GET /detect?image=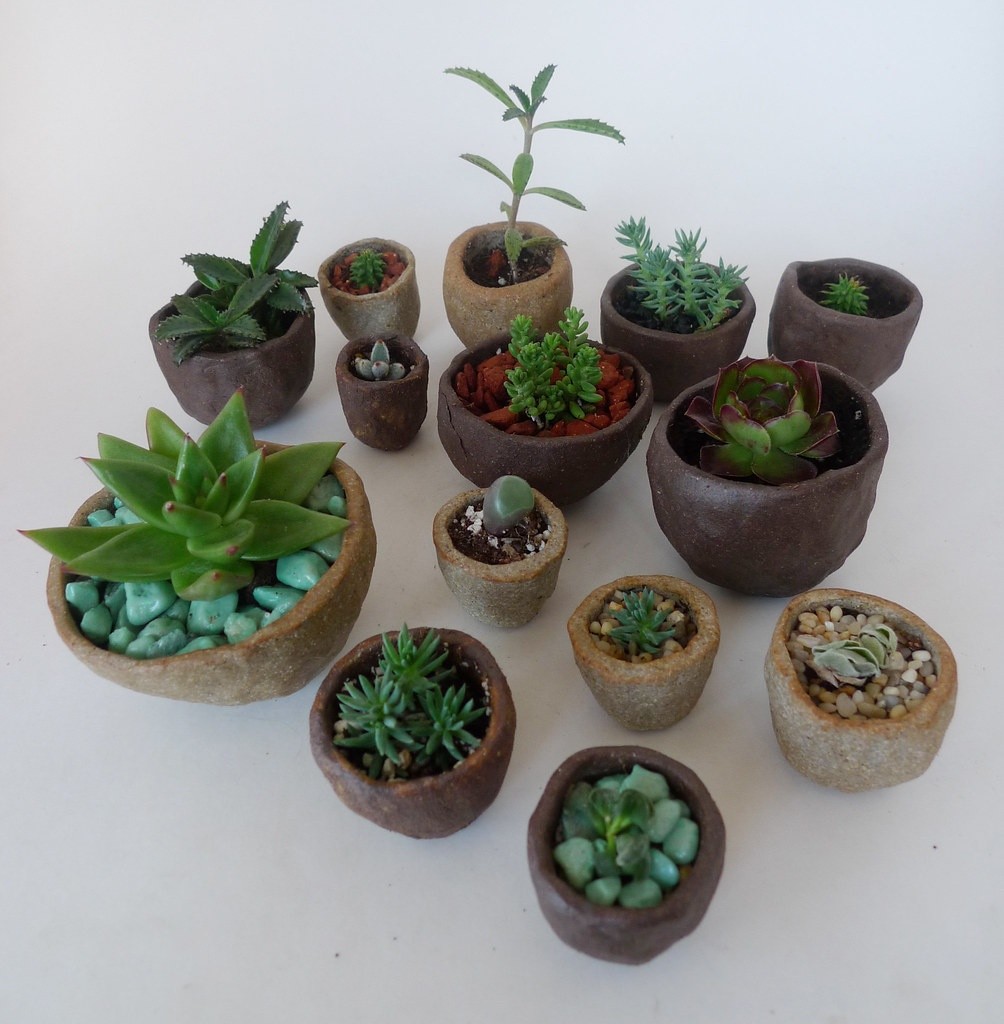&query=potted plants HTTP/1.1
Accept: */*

[435,308,654,509]
[768,257,923,396]
[311,626,515,840]
[598,216,757,403]
[763,589,959,792]
[148,201,320,429]
[645,353,888,601]
[566,574,722,733]
[442,66,626,348]
[318,237,420,346]
[335,332,430,451]
[15,387,378,706]
[526,743,728,965]
[435,473,568,632]
